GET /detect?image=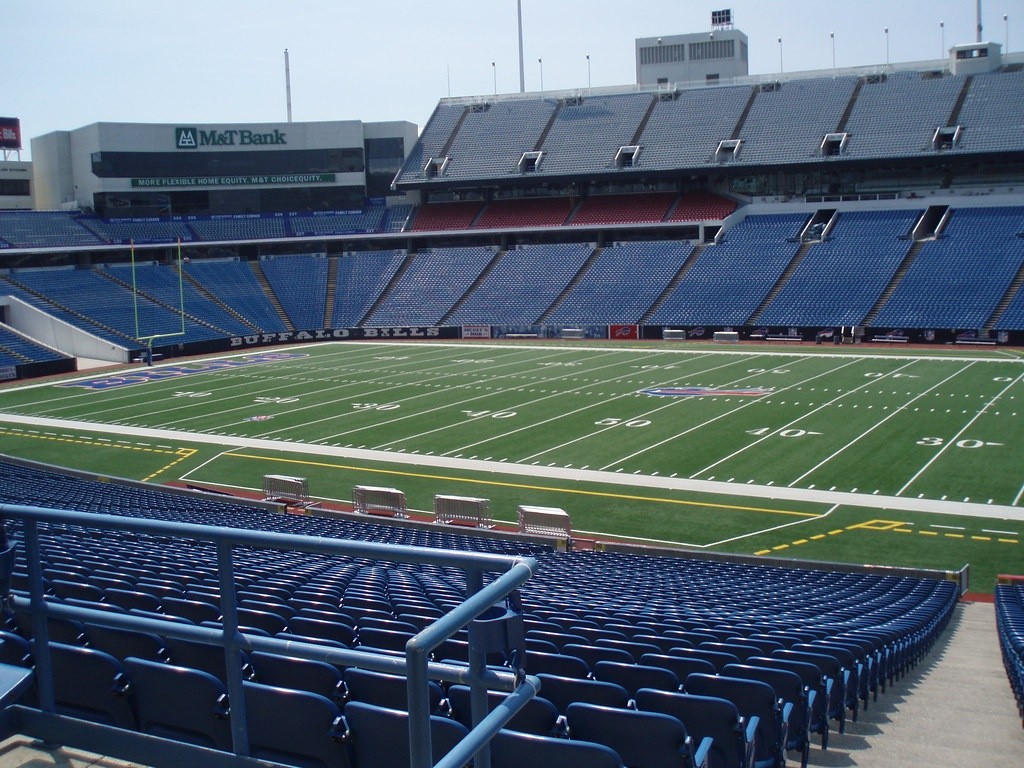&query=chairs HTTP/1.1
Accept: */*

[0,191,1024,768]
